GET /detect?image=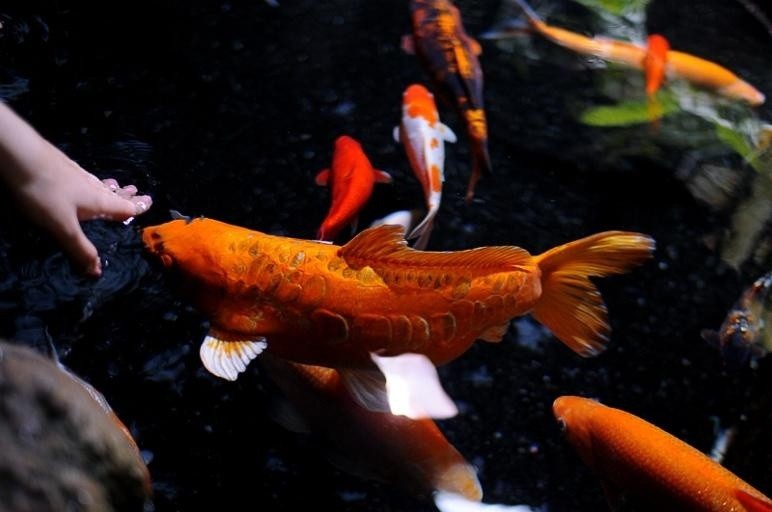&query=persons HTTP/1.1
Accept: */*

[0,103,153,275]
